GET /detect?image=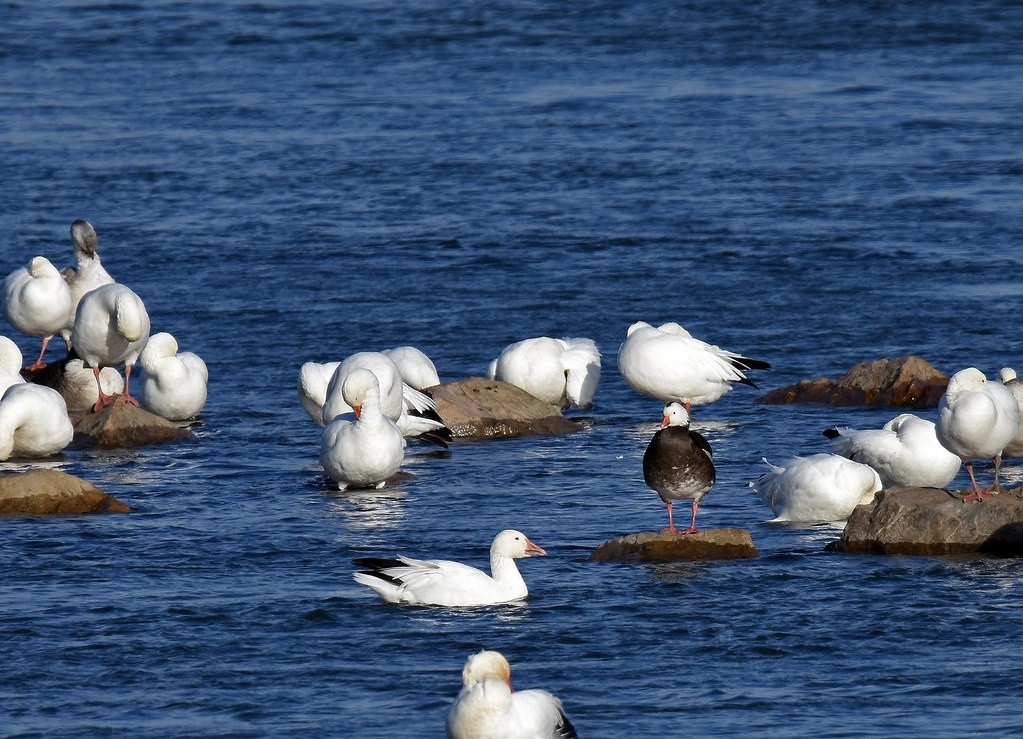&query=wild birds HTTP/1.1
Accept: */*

[741,366,1023,521]
[643,401,716,534]
[445,649,579,739]
[1,219,208,463]
[616,320,771,415]
[297,346,453,491]
[487,337,602,413]
[349,529,547,607]
